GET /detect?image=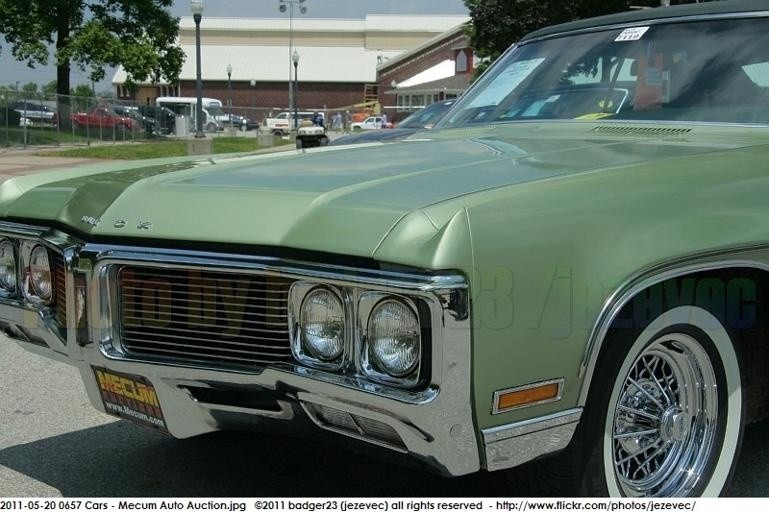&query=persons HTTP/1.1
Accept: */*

[376,49,384,64]
[310,110,353,134]
[381,111,388,129]
